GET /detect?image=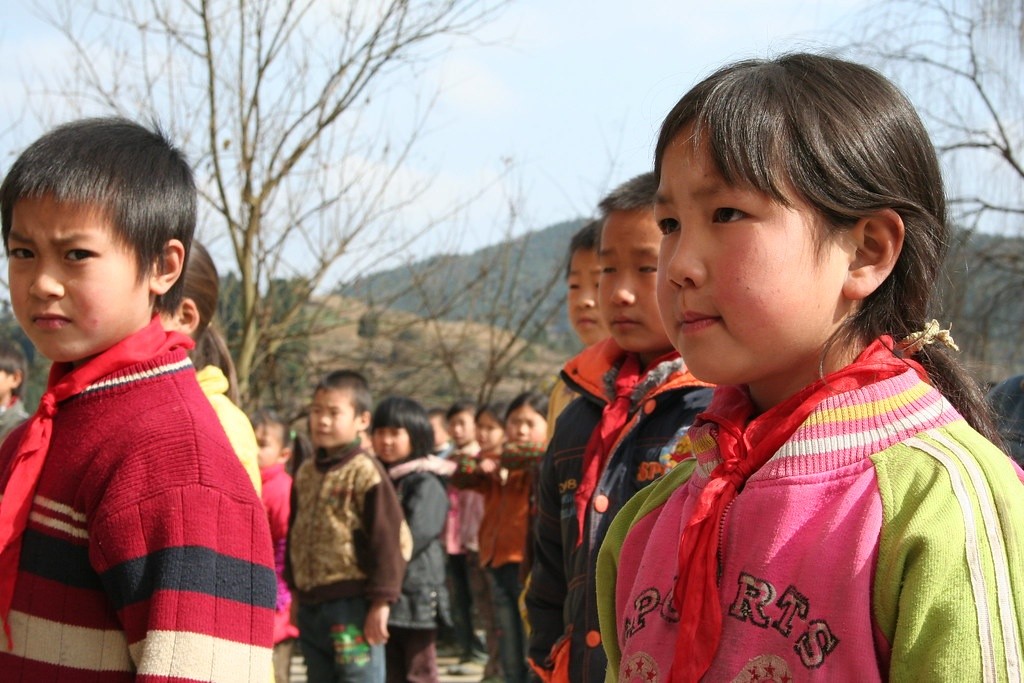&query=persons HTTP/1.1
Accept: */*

[523,171,718,683]
[0,115,278,683]
[0,214,602,683]
[592,52,1024,683]
